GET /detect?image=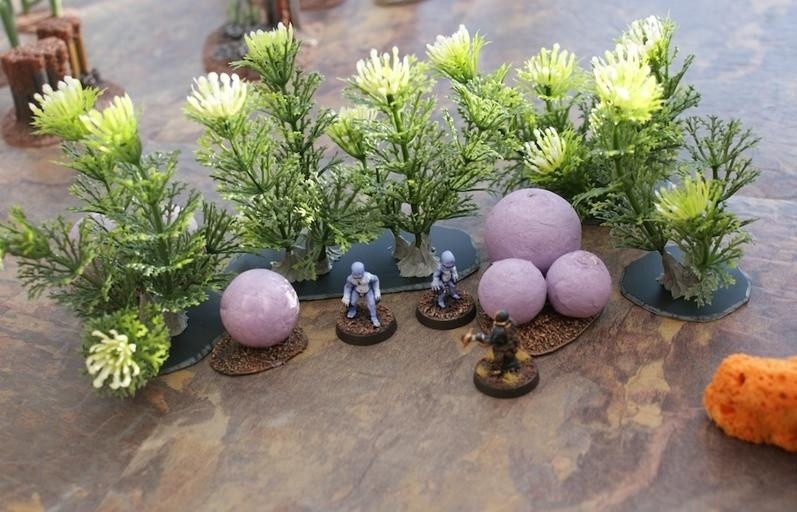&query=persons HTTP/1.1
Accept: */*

[338,260,382,328]
[429,248,463,309]
[457,309,521,379]
